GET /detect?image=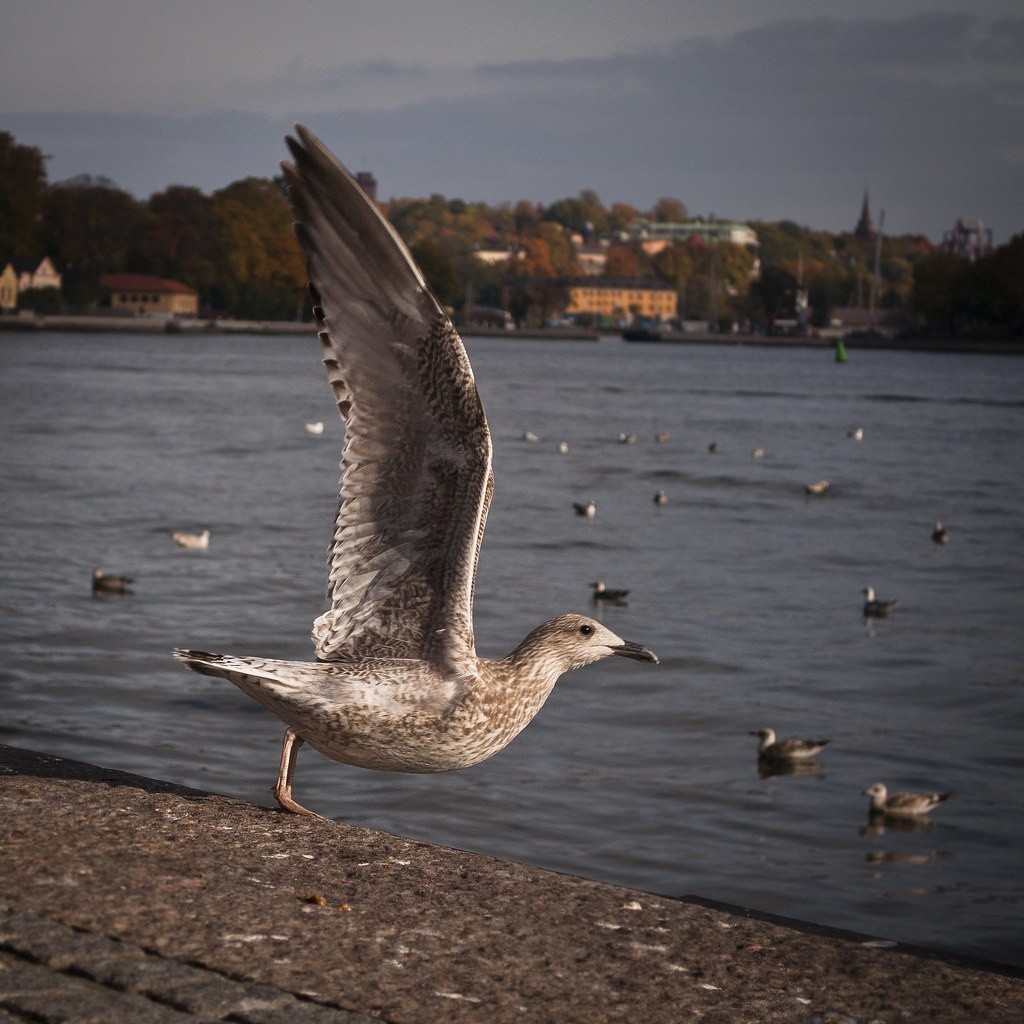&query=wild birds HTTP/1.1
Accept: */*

[90,122,957,823]
